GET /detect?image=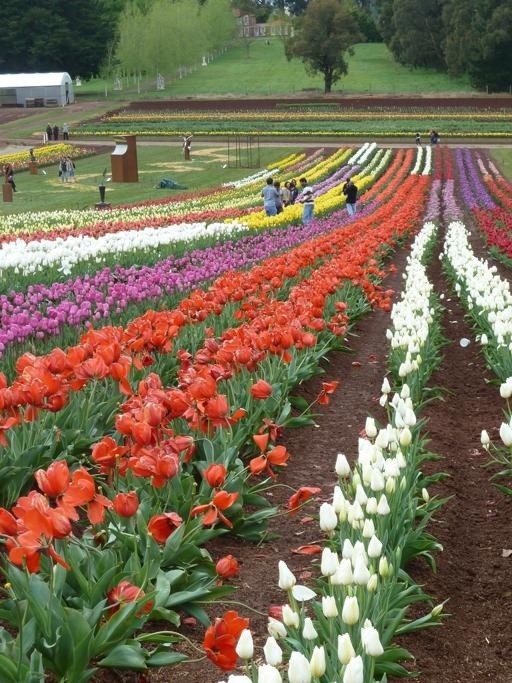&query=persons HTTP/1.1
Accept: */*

[261,177,297,216]
[342,178,349,191]
[344,182,358,216]
[416,131,440,145]
[184,132,194,162]
[3,122,76,192]
[300,178,314,222]
